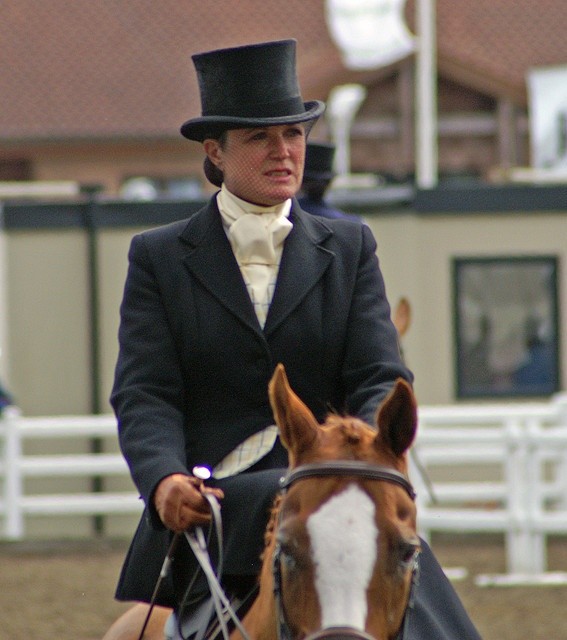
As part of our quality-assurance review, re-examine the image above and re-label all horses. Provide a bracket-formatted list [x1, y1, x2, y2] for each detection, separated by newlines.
[98, 361, 421, 640]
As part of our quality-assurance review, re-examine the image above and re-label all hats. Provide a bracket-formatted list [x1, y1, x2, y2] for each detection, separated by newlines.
[180, 38, 327, 143]
[303, 142, 338, 180]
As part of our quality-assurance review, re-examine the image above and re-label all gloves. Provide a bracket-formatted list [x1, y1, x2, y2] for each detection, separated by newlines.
[153, 473, 225, 532]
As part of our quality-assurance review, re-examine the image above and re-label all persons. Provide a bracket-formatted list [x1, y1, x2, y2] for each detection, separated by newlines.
[109, 37, 482, 639]
